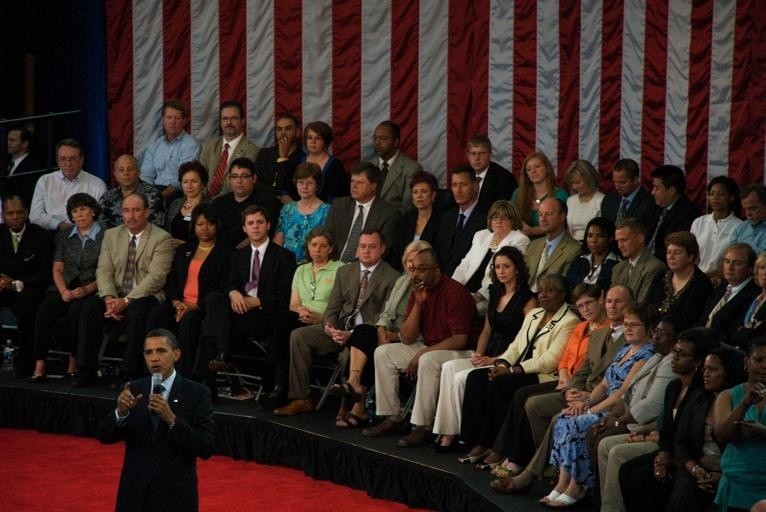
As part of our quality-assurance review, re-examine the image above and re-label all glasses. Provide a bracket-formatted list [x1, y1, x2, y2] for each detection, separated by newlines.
[409, 266, 435, 273]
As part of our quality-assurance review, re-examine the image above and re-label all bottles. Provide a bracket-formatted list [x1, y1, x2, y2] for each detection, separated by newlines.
[2, 340, 16, 373]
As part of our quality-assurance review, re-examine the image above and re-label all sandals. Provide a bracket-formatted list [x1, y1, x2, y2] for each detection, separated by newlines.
[328, 381, 589, 506]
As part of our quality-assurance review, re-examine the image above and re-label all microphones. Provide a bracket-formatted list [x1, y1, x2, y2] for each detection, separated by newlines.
[149, 372, 164, 412]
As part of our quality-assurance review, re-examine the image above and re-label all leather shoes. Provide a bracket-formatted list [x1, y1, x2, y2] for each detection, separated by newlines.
[65, 369, 81, 386]
[29, 374, 47, 383]
[208, 353, 236, 372]
[272, 399, 315, 416]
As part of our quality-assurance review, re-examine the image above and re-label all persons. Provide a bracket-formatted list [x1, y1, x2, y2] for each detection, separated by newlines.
[1, 101, 766, 512]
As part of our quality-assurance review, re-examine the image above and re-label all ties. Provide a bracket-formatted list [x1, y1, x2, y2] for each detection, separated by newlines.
[7, 161, 15, 176]
[12, 235, 19, 253]
[207, 143, 230, 199]
[245, 250, 261, 293]
[340, 205, 365, 262]
[151, 386, 165, 433]
[377, 161, 389, 197]
[123, 236, 137, 295]
[345, 271, 369, 329]
